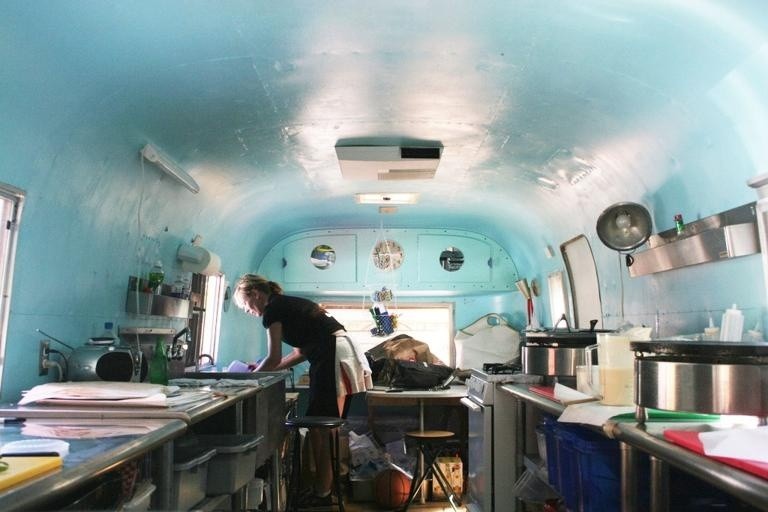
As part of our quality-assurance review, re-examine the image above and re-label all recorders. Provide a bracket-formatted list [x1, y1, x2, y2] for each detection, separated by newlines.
[67, 337, 149, 383]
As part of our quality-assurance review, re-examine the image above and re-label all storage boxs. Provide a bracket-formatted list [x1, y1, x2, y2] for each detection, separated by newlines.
[531, 413, 738, 511]
[432, 456, 464, 502]
[350, 480, 376, 503]
[53, 433, 264, 512]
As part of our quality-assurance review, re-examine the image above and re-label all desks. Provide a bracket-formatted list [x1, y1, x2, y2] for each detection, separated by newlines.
[366, 385, 469, 506]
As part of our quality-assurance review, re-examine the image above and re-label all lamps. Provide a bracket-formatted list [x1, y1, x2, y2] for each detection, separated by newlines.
[595, 201, 653, 252]
[138, 143, 200, 194]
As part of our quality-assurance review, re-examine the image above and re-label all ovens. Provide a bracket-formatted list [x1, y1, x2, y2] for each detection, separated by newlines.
[461, 396, 535, 512]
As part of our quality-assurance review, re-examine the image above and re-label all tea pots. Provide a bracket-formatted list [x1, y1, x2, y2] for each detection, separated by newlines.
[583, 328, 653, 407]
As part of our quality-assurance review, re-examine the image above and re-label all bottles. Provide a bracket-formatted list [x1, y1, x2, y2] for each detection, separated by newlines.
[102, 321, 120, 346]
[674, 213, 688, 240]
[146, 258, 167, 293]
[705, 301, 765, 344]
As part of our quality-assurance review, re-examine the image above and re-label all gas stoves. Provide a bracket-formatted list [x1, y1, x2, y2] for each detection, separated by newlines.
[465, 362, 536, 405]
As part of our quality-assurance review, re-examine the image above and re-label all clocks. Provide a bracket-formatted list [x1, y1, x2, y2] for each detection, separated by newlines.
[372, 239, 404, 271]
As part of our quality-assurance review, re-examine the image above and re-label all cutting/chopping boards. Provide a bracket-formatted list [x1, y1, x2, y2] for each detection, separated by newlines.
[0, 456, 63, 491]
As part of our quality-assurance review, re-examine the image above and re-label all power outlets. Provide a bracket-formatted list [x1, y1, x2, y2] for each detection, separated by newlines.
[38, 340, 50, 376]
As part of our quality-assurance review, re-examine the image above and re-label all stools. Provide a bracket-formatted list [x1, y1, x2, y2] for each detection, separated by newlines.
[284, 415, 346, 511]
[400, 430, 462, 512]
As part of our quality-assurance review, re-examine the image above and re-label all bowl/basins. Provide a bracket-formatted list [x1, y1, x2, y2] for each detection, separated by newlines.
[228, 360, 252, 373]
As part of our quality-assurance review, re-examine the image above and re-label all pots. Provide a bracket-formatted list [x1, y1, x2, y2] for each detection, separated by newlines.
[519, 327, 613, 378]
[627, 340, 768, 430]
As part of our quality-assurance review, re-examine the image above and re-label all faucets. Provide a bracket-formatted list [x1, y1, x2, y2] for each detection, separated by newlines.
[173, 327, 193, 360]
[196, 354, 214, 373]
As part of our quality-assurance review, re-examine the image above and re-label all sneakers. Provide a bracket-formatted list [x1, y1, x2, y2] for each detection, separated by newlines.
[298, 493, 332, 506]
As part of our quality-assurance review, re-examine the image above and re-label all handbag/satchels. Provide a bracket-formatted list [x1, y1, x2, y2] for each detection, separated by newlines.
[453, 314, 522, 371]
[387, 358, 457, 391]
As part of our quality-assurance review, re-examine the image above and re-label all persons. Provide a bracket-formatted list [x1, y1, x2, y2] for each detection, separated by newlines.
[233, 275, 374, 508]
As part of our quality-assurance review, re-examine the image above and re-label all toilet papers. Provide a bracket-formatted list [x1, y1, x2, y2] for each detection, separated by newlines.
[181, 245, 221, 275]
